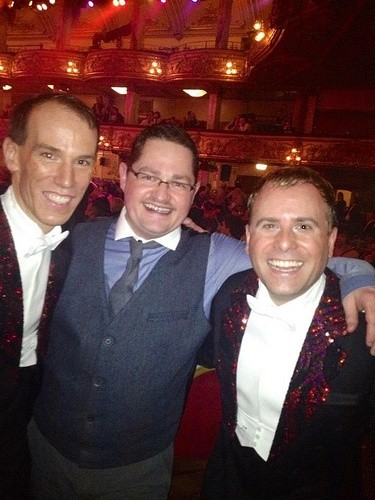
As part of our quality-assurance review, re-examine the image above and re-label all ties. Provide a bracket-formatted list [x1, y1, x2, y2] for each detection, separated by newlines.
[108, 240, 162, 316]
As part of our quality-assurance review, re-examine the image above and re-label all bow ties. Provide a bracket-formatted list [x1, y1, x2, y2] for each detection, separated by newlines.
[247, 294, 306, 331]
[23, 230, 70, 258]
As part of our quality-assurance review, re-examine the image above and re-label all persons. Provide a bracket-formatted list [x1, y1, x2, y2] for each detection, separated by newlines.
[0, 94, 101, 500]
[336, 191, 361, 234]
[194, 181, 247, 240]
[274, 113, 294, 135]
[27, 126, 375, 500]
[185, 110, 198, 126]
[89, 96, 124, 123]
[194, 167, 375, 500]
[138, 109, 162, 128]
[237, 114, 257, 133]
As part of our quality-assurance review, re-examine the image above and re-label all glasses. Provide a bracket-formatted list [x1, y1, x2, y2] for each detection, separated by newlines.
[128, 169, 196, 194]
